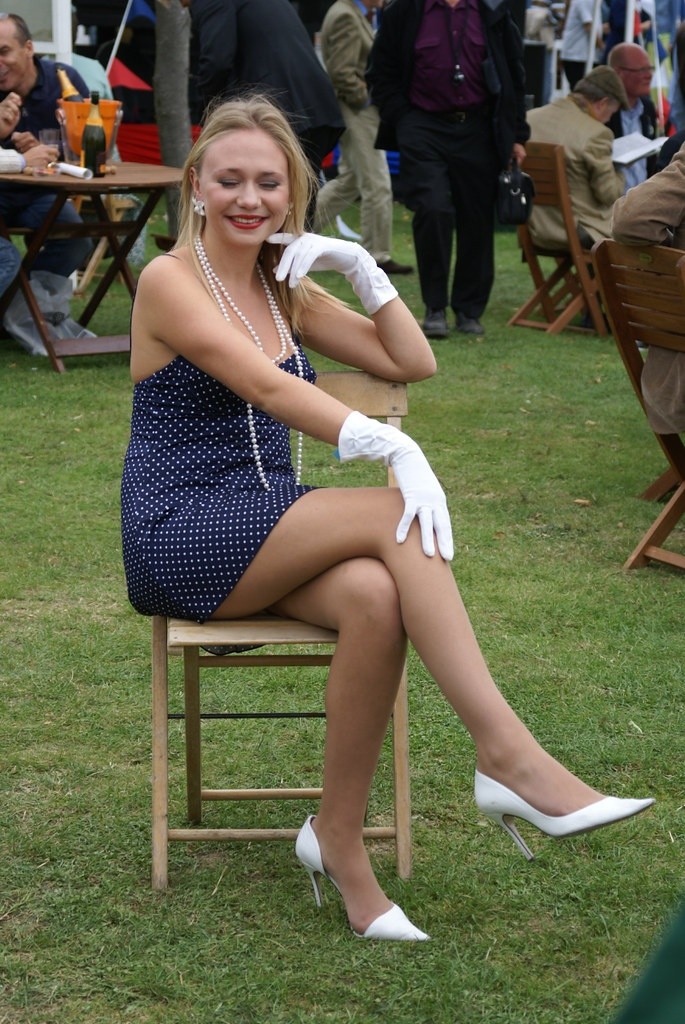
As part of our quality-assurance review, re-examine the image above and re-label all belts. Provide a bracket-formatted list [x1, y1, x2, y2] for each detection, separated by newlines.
[406, 102, 490, 125]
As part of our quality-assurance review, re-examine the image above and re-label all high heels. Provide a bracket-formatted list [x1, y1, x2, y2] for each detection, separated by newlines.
[295, 815, 431, 942]
[474, 769, 656, 863]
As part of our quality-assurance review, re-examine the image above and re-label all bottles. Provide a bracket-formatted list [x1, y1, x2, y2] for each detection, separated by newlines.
[80, 90, 106, 177]
[56, 66, 84, 104]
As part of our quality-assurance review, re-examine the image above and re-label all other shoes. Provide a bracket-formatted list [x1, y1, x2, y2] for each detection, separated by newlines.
[583, 310, 609, 329]
[454, 312, 485, 337]
[424, 308, 448, 337]
[377, 260, 414, 275]
[634, 340, 648, 348]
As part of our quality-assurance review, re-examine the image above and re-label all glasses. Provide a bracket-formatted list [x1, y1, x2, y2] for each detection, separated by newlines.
[615, 65, 654, 74]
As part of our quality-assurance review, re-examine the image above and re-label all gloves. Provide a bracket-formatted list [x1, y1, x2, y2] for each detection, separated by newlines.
[265, 230, 398, 315]
[337, 412, 455, 561]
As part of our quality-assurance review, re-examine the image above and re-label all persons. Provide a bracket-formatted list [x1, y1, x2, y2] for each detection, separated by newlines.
[525, 0, 651, 91]
[364, 0, 531, 337]
[318, 0, 412, 275]
[118, 96, 656, 941]
[176, 0, 348, 232]
[605, 44, 660, 196]
[516, 63, 628, 329]
[0, 10, 123, 358]
[610, 23, 685, 436]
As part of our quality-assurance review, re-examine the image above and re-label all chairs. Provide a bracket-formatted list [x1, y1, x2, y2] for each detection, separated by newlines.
[505, 142, 608, 335]
[149, 370, 412, 892]
[590, 238, 685, 580]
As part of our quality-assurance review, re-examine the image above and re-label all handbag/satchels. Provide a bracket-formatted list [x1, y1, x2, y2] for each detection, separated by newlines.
[497, 161, 535, 226]
[3, 270, 97, 356]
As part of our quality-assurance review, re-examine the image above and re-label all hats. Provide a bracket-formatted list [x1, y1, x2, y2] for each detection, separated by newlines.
[585, 65, 631, 113]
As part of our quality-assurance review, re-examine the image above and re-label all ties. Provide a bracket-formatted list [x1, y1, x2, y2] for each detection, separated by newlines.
[366, 11, 373, 26]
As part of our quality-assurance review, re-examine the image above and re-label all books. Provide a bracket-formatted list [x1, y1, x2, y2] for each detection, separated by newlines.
[608, 131, 669, 165]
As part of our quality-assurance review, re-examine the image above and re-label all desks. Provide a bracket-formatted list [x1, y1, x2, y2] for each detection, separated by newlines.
[1, 161, 185, 373]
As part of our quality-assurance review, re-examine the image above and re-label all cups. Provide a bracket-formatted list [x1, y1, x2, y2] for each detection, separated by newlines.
[39, 128, 60, 165]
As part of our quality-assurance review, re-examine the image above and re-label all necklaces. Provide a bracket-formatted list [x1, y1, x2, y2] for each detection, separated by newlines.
[194, 234, 308, 491]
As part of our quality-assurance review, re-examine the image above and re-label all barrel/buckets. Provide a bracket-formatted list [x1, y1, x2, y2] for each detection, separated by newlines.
[56, 96, 122, 167]
